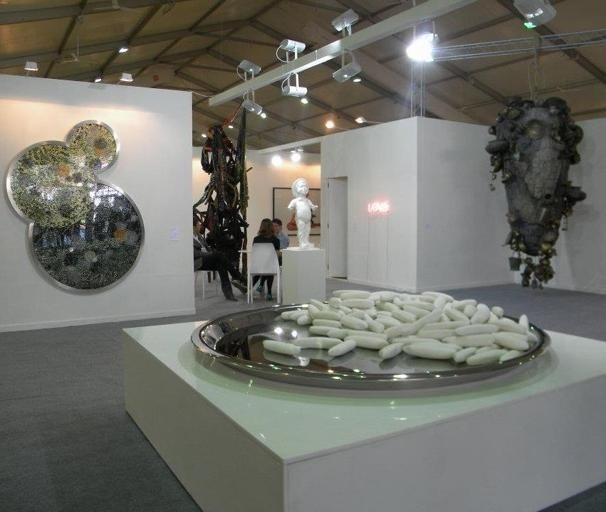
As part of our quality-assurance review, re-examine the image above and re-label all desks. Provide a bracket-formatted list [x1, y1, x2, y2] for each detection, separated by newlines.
[238, 249, 284, 299]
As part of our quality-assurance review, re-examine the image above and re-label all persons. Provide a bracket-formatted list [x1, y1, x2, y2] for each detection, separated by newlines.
[193, 216, 288, 301]
[287, 176, 318, 247]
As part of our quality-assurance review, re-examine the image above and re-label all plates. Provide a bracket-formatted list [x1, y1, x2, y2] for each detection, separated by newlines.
[191, 301, 551, 387]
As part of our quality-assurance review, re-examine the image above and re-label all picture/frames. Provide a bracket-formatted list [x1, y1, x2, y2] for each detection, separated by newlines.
[273, 187, 321, 236]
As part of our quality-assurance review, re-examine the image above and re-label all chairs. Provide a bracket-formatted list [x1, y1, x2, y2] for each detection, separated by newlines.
[247, 242, 281, 304]
[195, 270, 222, 301]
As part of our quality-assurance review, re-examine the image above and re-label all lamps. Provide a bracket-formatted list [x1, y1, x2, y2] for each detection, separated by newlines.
[208, 0, 489, 119]
[94, 70, 105, 83]
[118, 44, 130, 54]
[355, 116, 385, 124]
[513, 0, 557, 29]
[289, 145, 304, 163]
[24, 62, 39, 77]
[120, 73, 134, 83]
